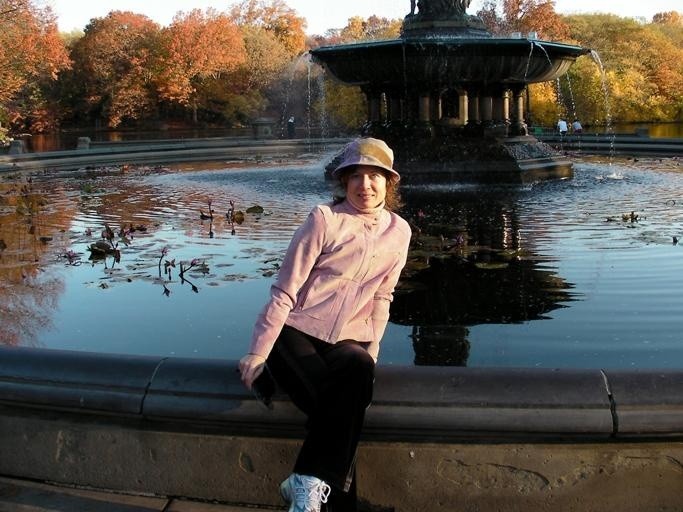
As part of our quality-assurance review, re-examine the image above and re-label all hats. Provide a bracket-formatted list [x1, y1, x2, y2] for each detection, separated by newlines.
[330, 136, 402, 186]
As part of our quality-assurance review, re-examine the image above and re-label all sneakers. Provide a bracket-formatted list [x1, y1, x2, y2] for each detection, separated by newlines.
[278, 470, 332, 512]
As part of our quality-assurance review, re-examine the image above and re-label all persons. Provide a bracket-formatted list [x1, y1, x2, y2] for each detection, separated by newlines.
[234, 134, 412, 512]
[286, 115, 296, 139]
[557, 118, 567, 142]
[571, 119, 584, 135]
